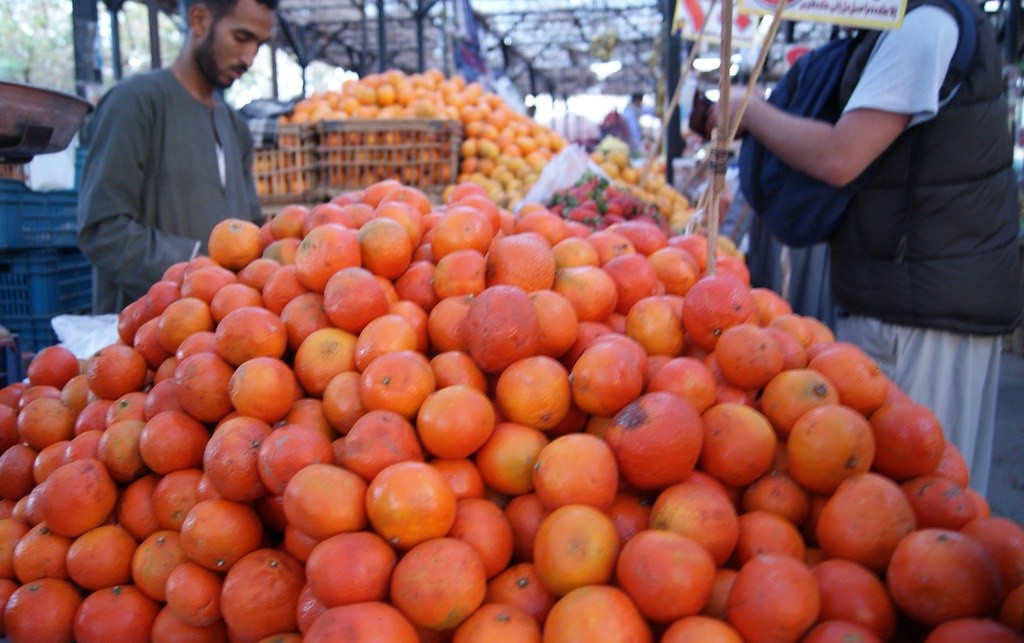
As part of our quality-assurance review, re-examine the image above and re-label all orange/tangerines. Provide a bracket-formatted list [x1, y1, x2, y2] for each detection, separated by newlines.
[0, 338, 1024, 643]
[554, 218, 833, 351]
[113, 178, 553, 352]
[589, 150, 742, 263]
[253, 67, 562, 205]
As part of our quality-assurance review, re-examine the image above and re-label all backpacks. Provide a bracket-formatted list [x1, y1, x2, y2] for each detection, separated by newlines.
[737, 1, 979, 250]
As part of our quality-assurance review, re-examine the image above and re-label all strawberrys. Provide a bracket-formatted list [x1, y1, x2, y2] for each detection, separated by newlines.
[547, 170, 666, 235]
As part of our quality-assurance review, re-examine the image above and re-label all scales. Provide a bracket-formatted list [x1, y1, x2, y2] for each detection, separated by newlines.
[0, 81, 95, 164]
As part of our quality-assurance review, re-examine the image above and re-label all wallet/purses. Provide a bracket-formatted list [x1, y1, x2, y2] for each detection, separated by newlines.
[690, 89, 746, 140]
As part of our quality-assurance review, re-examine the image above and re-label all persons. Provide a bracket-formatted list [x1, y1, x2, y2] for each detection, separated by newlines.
[710, 0, 1024, 501]
[621, 91, 655, 156]
[76, 0, 280, 316]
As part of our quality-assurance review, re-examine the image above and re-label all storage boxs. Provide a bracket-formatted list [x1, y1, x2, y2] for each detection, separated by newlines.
[0, 178, 79, 250]
[0, 318, 63, 384]
[0, 248, 92, 320]
[248, 123, 319, 214]
[316, 118, 463, 198]
